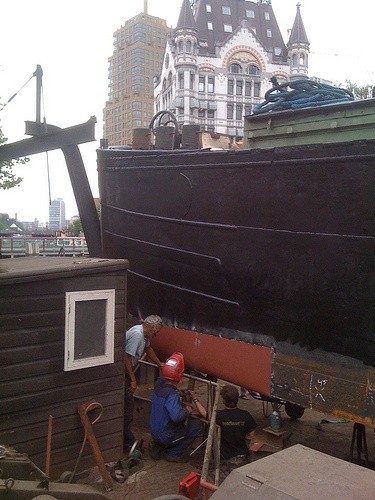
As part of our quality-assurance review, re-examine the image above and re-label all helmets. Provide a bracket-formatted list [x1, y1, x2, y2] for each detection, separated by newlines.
[162, 352, 184, 382]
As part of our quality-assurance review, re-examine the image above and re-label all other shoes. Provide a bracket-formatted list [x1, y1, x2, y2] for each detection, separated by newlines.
[150, 447, 161, 460]
[166, 455, 188, 463]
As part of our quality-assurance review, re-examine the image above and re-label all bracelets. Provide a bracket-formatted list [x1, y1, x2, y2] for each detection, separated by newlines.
[193, 398, 199, 404]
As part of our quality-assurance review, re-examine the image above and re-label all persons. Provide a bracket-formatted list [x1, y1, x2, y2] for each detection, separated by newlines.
[150, 351, 204, 461]
[111, 315, 162, 453]
[188, 385, 257, 461]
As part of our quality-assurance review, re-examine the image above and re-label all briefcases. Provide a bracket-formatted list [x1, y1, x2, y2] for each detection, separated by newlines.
[178, 471, 201, 498]
